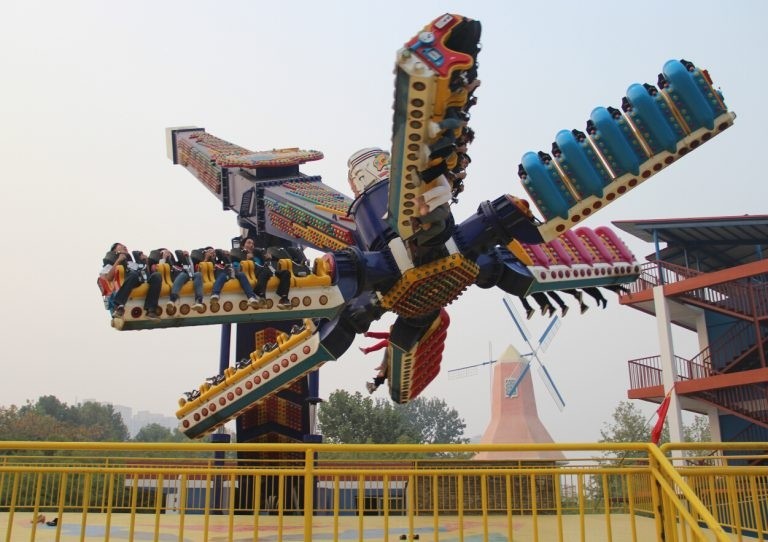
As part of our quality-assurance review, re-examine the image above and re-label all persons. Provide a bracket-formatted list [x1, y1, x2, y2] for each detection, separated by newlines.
[521, 284, 632, 320]
[100, 237, 292, 321]
[359, 324, 395, 395]
[412, 100, 473, 251]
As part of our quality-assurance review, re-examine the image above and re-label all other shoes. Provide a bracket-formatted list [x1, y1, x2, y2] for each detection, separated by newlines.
[526, 309, 535, 320]
[602, 299, 607, 309]
[167, 301, 174, 312]
[626, 290, 633, 302]
[597, 297, 601, 306]
[364, 332, 368, 337]
[540, 304, 548, 316]
[146, 311, 160, 322]
[112, 305, 125, 317]
[358, 347, 368, 355]
[259, 298, 267, 305]
[421, 145, 431, 164]
[428, 121, 441, 138]
[367, 383, 376, 394]
[416, 218, 424, 227]
[283, 296, 291, 306]
[411, 236, 420, 248]
[373, 378, 384, 385]
[411, 169, 422, 186]
[549, 308, 556, 317]
[561, 306, 569, 317]
[211, 296, 219, 303]
[248, 298, 259, 306]
[580, 304, 587, 313]
[191, 300, 204, 310]
